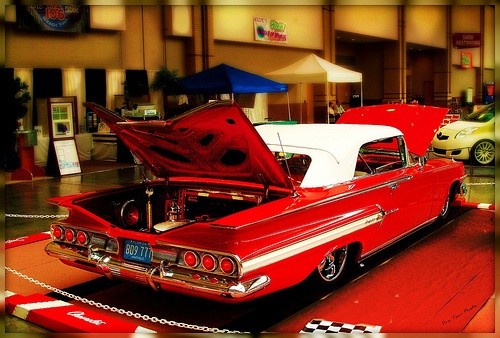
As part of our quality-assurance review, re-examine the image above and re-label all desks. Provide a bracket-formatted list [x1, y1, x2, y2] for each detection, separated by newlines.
[33, 133, 93, 167]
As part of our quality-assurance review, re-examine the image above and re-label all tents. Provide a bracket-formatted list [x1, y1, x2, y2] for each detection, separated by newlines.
[264, 54, 362, 124]
[163, 63, 291, 121]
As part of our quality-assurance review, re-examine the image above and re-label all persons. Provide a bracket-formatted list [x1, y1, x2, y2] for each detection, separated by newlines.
[329, 99, 345, 123]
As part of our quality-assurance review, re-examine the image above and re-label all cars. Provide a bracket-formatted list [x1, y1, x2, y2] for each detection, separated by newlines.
[431, 103, 496, 165]
[45, 100, 470, 310]
